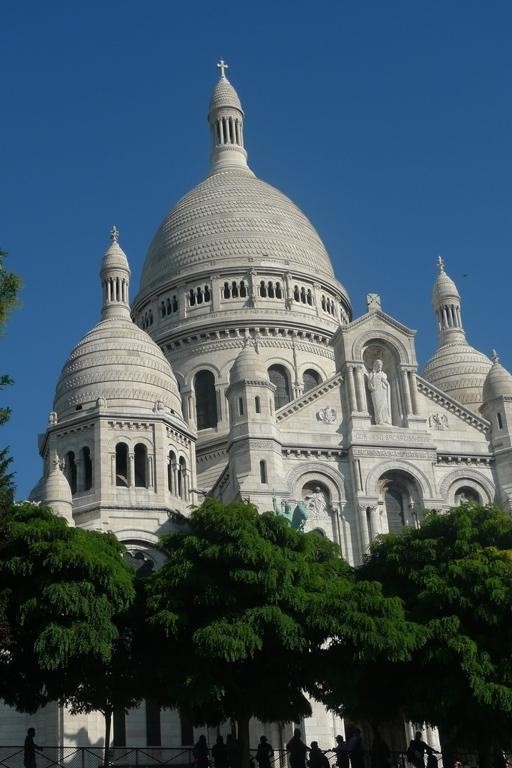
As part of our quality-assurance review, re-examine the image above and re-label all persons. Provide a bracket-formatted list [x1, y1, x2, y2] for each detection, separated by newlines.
[367, 359, 393, 425]
[193, 729, 461, 768]
[24, 728, 42, 768]
[306, 487, 326, 514]
